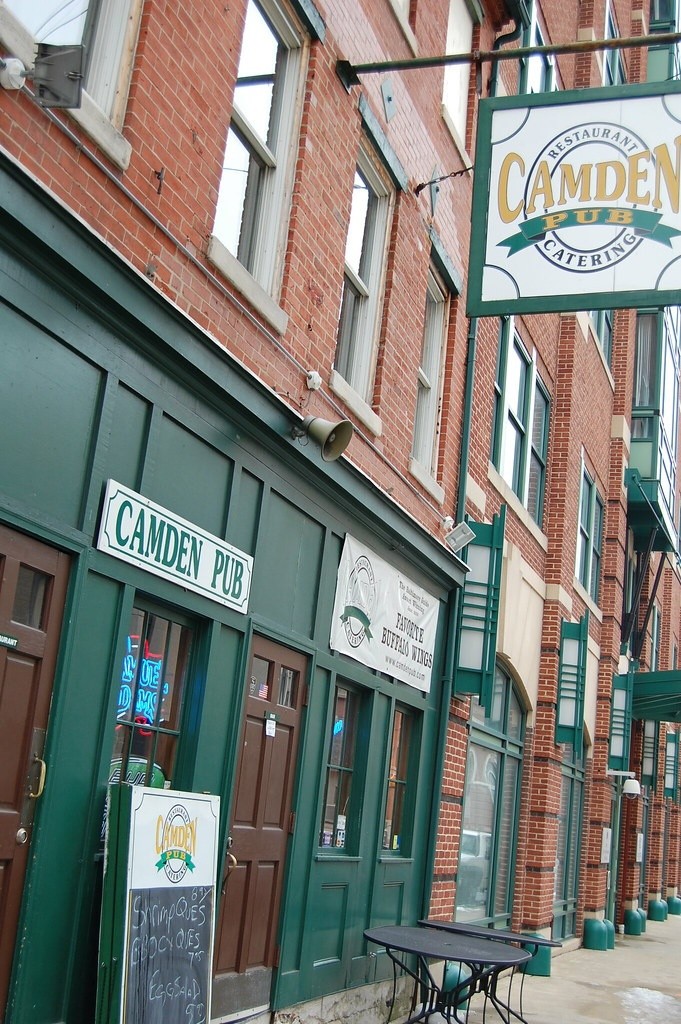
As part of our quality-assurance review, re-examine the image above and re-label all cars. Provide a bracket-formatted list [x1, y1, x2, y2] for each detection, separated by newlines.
[456, 829, 494, 907]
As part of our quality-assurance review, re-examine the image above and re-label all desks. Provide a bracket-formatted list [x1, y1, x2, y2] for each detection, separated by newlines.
[418, 918, 562, 1024]
[363, 924, 532, 1024]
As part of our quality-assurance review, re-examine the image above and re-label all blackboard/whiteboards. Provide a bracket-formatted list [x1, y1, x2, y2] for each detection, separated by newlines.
[123, 885, 215, 1024]
